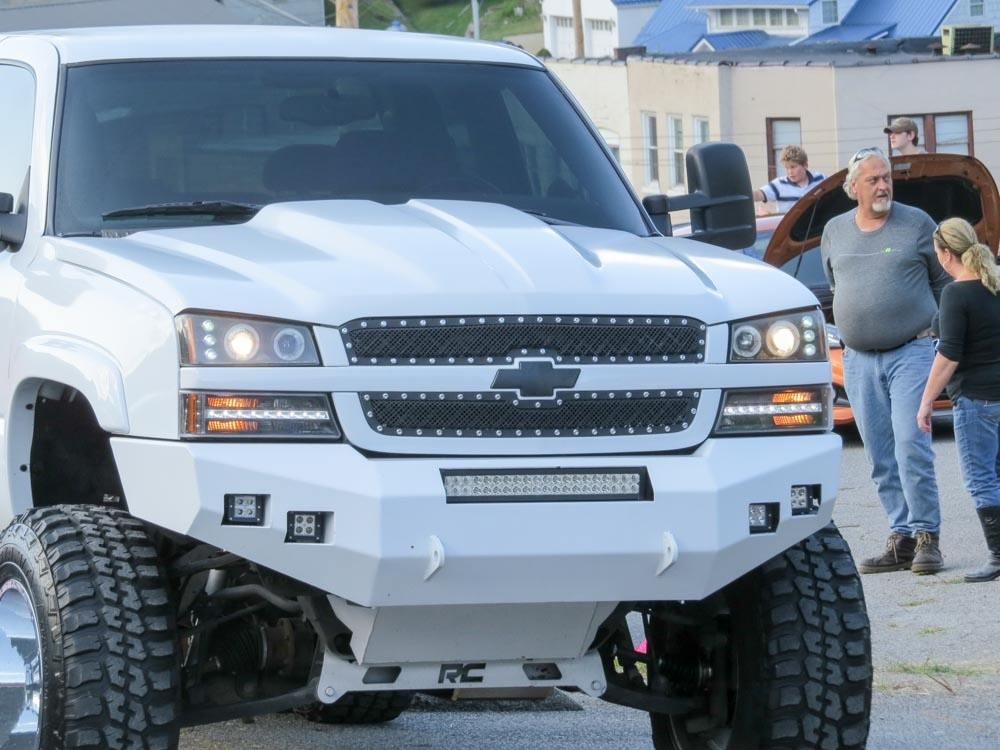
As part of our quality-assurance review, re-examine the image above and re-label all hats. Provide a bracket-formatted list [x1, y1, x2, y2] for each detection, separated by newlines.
[884, 117, 918, 134]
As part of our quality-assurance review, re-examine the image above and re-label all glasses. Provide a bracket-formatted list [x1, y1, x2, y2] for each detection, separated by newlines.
[854, 147, 884, 162]
[936, 219, 951, 248]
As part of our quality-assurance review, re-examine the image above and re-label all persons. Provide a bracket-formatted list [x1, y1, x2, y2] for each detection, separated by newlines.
[883, 117, 930, 154]
[750, 145, 826, 215]
[916, 217, 1000, 582]
[820, 145, 955, 574]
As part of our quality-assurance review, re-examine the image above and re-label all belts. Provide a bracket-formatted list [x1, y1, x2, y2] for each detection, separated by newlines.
[867, 329, 928, 352]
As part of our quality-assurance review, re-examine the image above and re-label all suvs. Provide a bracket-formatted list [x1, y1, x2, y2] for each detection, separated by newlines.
[0, 25, 872, 750]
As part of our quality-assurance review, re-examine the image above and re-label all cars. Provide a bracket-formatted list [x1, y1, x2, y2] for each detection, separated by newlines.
[672, 153, 1000, 427]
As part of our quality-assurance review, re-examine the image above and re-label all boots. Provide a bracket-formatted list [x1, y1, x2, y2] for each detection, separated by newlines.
[860, 533, 917, 573]
[911, 530, 944, 574]
[964, 505, 1000, 581]
